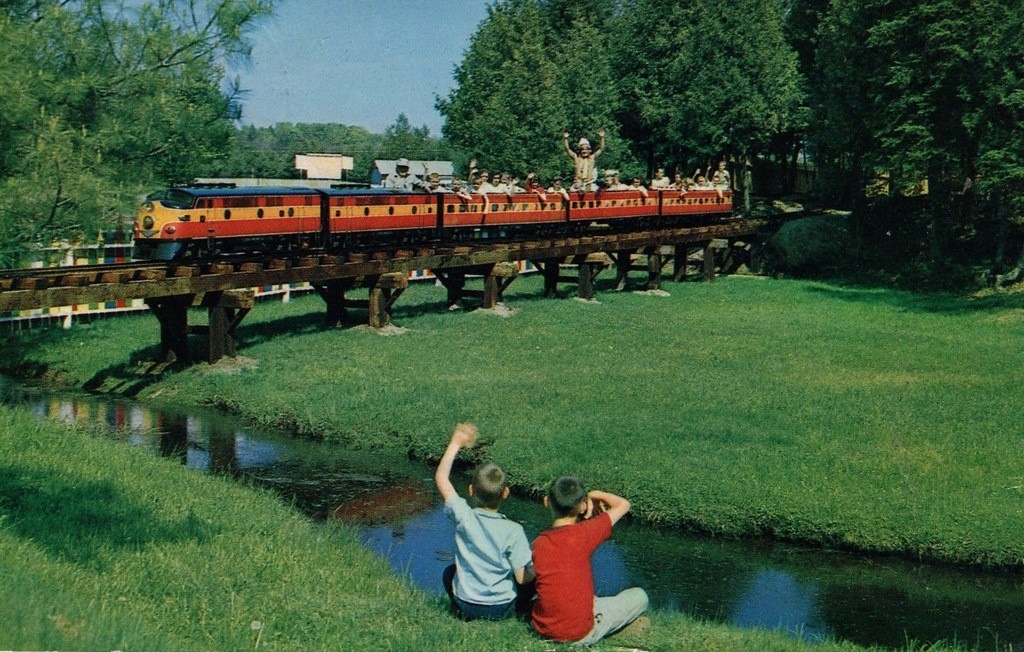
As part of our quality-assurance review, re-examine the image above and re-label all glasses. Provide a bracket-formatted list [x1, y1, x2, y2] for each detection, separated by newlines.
[493, 177, 500, 179]
[432, 181, 439, 184]
[481, 176, 489, 178]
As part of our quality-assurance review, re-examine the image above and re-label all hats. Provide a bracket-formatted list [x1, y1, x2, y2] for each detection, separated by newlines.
[578, 137, 590, 148]
[395, 157, 409, 166]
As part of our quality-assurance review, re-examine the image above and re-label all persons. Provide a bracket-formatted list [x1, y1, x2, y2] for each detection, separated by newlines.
[530, 475, 649, 647]
[564, 127, 605, 192]
[435, 420, 534, 621]
[385, 158, 730, 214]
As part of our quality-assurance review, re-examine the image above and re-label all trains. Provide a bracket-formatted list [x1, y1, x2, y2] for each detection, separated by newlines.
[129, 177, 735, 266]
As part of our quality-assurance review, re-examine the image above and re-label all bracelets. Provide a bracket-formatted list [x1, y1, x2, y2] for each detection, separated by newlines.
[600, 136, 604, 138]
[564, 138, 568, 139]
